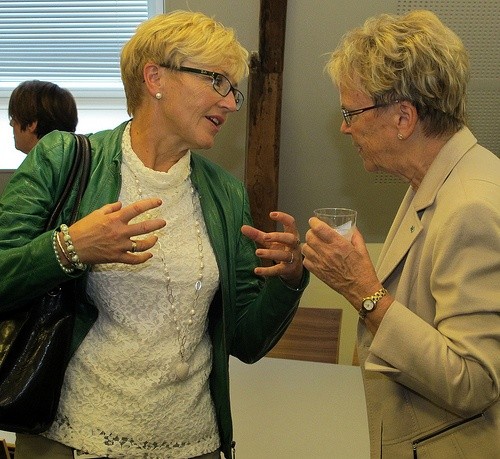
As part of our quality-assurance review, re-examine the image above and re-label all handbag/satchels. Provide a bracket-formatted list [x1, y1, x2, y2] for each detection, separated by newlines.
[0, 133, 99, 435]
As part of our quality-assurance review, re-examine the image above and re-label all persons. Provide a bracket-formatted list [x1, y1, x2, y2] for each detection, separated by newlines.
[0, 11, 309, 458]
[7, 80, 78, 155]
[301, 10, 500, 458]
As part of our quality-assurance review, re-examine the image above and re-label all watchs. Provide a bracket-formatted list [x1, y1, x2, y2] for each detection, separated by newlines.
[358, 289, 388, 320]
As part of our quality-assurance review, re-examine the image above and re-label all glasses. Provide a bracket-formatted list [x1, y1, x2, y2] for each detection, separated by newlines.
[341, 99, 423, 126]
[142, 65, 244, 110]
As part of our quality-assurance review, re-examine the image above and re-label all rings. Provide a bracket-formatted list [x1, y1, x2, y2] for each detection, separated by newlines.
[287, 253, 294, 264]
[295, 239, 301, 246]
[130, 240, 137, 253]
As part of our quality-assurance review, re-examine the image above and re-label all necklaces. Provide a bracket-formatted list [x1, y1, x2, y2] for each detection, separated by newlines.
[122, 129, 205, 381]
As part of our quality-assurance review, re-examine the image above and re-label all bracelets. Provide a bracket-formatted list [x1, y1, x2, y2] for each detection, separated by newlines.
[53, 224, 86, 273]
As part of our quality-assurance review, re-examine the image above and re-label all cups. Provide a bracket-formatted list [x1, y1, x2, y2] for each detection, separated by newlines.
[313, 208, 358, 244]
